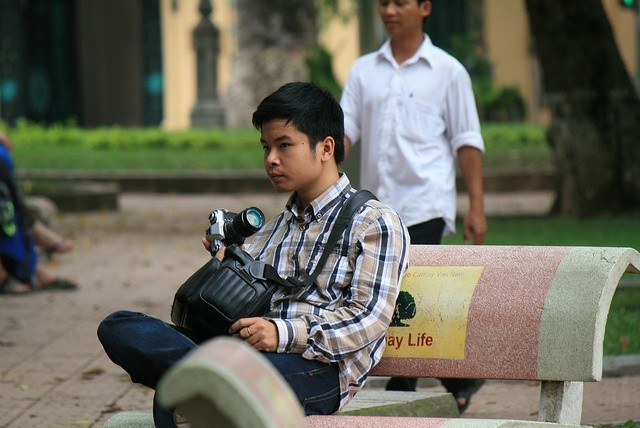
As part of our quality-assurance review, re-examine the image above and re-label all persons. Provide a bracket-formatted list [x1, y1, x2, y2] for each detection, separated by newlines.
[339, 0, 486, 415]
[1, 130, 80, 296]
[98, 81, 411, 416]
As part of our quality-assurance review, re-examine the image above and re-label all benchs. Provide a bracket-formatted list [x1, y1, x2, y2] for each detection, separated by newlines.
[102, 245, 639, 428]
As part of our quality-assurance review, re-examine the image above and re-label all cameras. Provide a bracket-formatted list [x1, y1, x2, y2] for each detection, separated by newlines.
[205, 206, 265, 257]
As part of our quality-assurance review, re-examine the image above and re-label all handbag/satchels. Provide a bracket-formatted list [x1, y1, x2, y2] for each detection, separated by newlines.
[171, 243, 280, 335]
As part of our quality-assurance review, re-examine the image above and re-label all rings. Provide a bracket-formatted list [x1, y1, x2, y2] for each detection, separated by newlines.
[246, 326, 253, 336]
